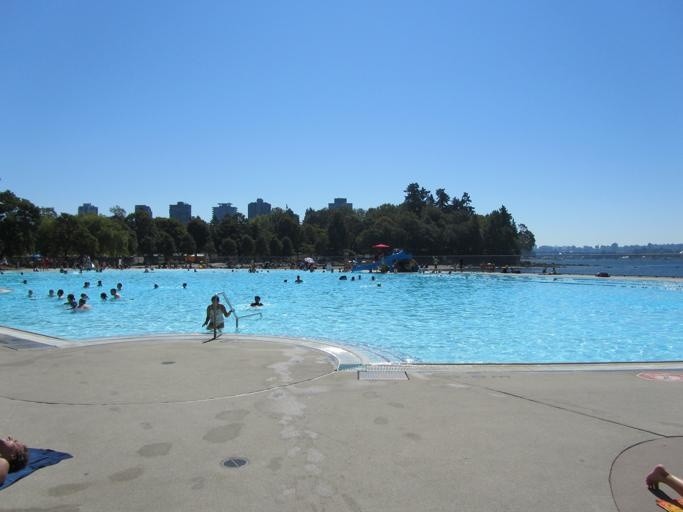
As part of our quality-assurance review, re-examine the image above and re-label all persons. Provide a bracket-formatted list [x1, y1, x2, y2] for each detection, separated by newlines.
[250, 295, 262, 306]
[27, 289, 32, 298]
[294, 275, 302, 284]
[200, 294, 235, 329]
[0, 434, 28, 489]
[644, 463, 682, 497]
[47, 280, 122, 313]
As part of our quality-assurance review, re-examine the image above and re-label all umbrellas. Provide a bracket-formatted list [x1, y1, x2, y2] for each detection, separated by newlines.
[304, 256, 315, 264]
[370, 243, 390, 249]
